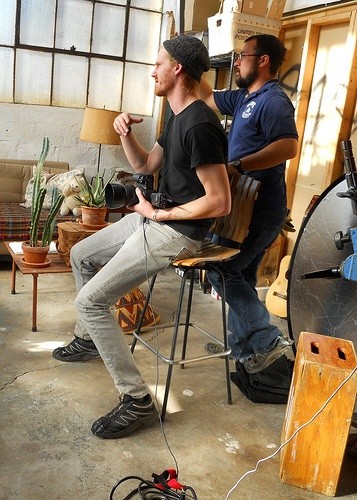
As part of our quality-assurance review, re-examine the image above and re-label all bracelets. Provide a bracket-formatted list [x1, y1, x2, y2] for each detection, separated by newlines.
[152, 209, 158, 221]
[117, 126, 132, 134]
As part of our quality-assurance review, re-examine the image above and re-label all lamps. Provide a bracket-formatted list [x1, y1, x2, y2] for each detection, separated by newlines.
[80, 105, 122, 189]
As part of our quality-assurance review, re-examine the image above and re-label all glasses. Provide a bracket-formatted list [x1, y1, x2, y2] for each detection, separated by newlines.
[239, 52, 264, 60]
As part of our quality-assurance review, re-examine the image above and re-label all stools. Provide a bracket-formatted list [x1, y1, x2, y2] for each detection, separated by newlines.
[131, 172, 261, 422]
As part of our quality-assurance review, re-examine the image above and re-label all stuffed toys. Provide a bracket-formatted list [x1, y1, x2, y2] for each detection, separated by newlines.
[60, 178, 84, 216]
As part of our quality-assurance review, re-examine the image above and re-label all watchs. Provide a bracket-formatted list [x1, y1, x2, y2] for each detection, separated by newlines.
[234, 159, 244, 173]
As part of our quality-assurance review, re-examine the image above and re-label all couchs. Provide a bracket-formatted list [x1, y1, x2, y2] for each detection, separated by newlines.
[0, 159, 82, 263]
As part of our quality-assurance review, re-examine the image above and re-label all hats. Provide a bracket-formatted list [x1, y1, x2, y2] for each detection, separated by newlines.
[163, 35, 210, 84]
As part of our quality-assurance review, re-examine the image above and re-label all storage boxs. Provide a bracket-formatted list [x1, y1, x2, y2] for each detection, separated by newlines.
[208, 11, 283, 57]
[281, 333, 357, 497]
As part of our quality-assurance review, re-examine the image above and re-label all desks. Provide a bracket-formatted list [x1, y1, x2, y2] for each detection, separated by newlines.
[4, 241, 74, 331]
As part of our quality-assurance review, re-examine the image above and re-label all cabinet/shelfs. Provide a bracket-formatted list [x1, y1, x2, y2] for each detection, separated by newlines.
[210, 51, 283, 136]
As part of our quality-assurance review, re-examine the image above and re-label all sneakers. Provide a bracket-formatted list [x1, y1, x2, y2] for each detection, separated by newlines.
[52, 333, 101, 361]
[245, 333, 293, 373]
[91, 394, 159, 439]
[205, 342, 235, 360]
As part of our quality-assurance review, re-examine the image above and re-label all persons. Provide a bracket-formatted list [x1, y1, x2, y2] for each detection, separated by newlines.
[195, 35, 300, 374]
[53, 35, 232, 440]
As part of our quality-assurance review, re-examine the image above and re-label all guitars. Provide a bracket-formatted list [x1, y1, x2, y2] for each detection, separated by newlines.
[264, 194, 325, 317]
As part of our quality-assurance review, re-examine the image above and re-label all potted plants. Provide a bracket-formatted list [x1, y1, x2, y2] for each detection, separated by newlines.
[74, 168, 117, 224]
[22, 136, 65, 263]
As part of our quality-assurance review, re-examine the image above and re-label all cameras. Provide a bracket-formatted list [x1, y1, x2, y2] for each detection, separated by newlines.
[105, 172, 173, 209]
